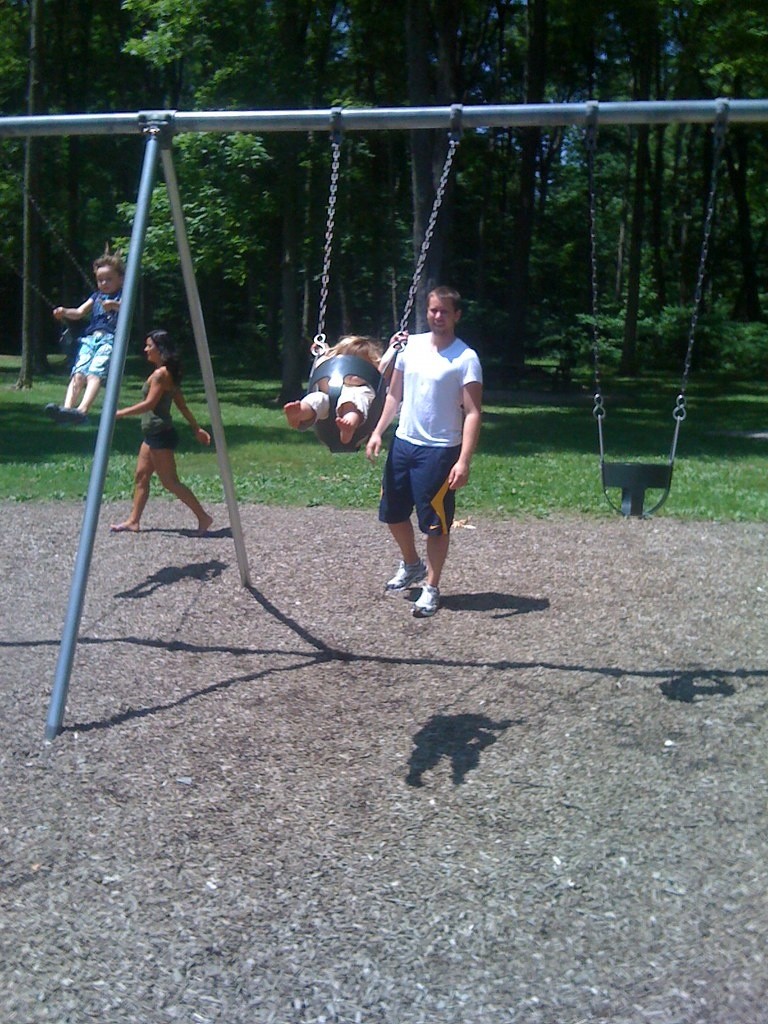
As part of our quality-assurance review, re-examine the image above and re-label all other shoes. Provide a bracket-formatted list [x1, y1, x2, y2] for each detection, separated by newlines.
[61, 406, 89, 424]
[44, 402, 65, 425]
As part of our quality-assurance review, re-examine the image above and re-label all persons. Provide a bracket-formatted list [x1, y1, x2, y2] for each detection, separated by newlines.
[284, 331, 409, 444]
[110, 332, 213, 536]
[46, 243, 123, 423]
[365, 286, 482, 617]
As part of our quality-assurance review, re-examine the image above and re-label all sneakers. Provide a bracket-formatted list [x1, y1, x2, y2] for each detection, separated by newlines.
[386, 558, 428, 591]
[411, 587, 440, 617]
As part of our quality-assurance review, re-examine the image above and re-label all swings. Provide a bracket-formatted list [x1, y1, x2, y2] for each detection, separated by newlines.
[306, 137, 461, 455]
[0, 152, 123, 388]
[586, 126, 727, 518]
